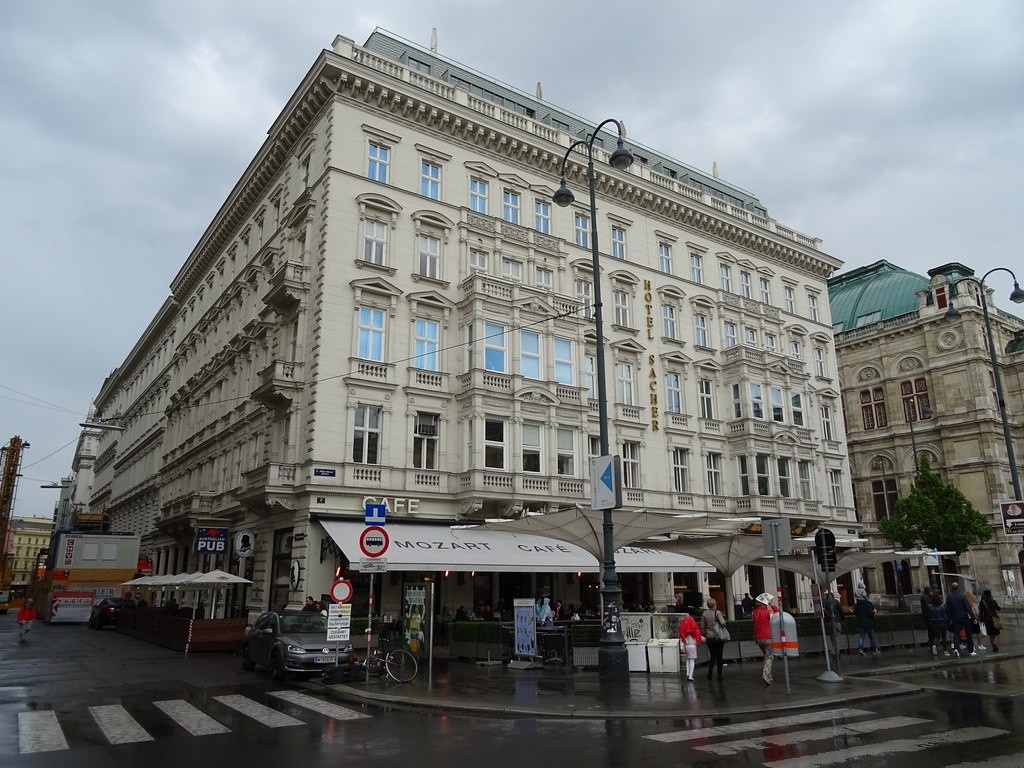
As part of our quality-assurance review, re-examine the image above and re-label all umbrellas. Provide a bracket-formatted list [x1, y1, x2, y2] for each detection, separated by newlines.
[119, 568, 254, 620]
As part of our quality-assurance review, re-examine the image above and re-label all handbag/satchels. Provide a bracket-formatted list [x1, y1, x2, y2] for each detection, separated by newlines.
[948, 621, 955, 633]
[713, 621, 731, 644]
[979, 622, 987, 636]
[992, 616, 1003, 630]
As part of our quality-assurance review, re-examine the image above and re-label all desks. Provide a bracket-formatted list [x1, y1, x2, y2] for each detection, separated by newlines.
[379, 622, 397, 644]
[536, 626, 572, 660]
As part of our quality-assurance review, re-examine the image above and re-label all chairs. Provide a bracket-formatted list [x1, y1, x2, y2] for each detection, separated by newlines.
[310, 621, 325, 632]
[378, 622, 394, 650]
[270, 618, 286, 633]
[502, 625, 566, 664]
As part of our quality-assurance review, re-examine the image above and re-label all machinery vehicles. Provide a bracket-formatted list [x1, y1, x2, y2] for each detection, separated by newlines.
[0, 436, 30, 614]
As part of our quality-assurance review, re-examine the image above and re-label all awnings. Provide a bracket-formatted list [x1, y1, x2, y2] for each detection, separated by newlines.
[307, 512, 718, 572]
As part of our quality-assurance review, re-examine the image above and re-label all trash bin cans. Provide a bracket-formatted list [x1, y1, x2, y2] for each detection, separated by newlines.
[769, 613, 799, 656]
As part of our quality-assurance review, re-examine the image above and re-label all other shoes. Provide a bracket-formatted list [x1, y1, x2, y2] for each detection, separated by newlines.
[21, 639, 26, 642]
[951, 643, 954, 649]
[992, 643, 999, 653]
[944, 651, 950, 656]
[859, 650, 865, 655]
[970, 652, 977, 655]
[959, 644, 966, 649]
[932, 645, 937, 655]
[717, 678, 723, 681]
[688, 676, 694, 681]
[954, 649, 959, 657]
[20, 633, 22, 639]
[873, 648, 881, 654]
[763, 678, 770, 686]
[978, 644, 986, 650]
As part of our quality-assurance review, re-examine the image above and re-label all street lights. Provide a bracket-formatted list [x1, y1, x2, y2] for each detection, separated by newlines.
[552, 119, 634, 678]
[944, 268, 1024, 586]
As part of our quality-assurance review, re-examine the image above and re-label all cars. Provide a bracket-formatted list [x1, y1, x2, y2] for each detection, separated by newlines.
[88, 598, 136, 629]
[245, 609, 353, 678]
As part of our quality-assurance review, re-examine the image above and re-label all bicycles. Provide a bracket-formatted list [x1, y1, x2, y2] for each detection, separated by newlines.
[354, 646, 418, 683]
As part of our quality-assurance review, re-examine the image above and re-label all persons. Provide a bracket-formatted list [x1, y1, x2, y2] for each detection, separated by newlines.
[17, 598, 36, 643]
[456, 596, 660, 625]
[678, 581, 1002, 685]
[306, 596, 318, 611]
